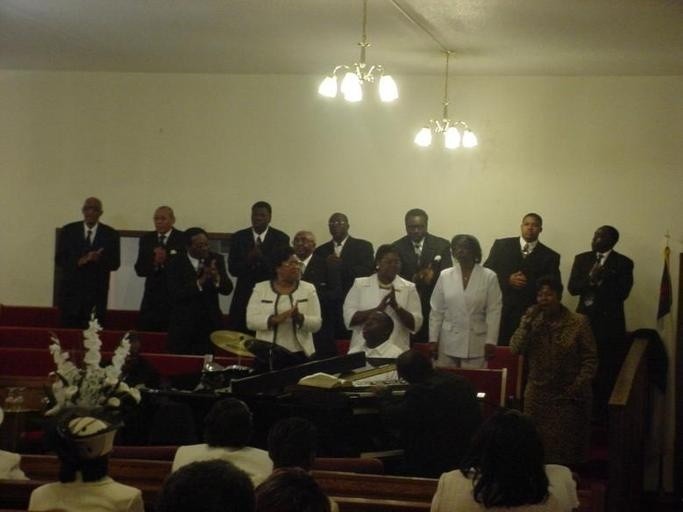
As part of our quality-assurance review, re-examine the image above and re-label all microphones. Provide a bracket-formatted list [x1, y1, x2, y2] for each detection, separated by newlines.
[525, 303, 542, 325]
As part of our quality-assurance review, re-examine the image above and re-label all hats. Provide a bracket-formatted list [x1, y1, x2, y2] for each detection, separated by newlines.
[56, 409, 119, 461]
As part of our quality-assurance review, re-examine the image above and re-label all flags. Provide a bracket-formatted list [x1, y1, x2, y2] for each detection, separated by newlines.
[657, 248, 673, 321]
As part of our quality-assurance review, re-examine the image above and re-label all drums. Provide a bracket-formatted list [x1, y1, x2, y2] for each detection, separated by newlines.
[201, 365, 253, 388]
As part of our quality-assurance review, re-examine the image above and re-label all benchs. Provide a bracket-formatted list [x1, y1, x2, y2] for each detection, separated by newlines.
[0, 298, 525, 511]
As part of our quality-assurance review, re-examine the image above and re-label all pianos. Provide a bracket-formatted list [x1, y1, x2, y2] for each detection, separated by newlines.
[297, 372, 411, 458]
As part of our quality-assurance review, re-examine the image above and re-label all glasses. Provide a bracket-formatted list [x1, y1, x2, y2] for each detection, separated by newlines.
[329, 220, 348, 226]
[279, 260, 304, 267]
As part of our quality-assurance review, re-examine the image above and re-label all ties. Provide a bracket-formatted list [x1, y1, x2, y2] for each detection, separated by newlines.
[159, 235, 166, 245]
[85, 230, 92, 247]
[256, 236, 262, 249]
[596, 254, 605, 265]
[414, 243, 423, 255]
[523, 244, 530, 254]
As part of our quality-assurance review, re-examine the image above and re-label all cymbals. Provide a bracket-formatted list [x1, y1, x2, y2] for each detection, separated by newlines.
[244, 339, 295, 366]
[210, 330, 257, 357]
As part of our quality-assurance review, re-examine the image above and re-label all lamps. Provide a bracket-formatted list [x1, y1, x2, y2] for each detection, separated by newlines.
[413, 51, 478, 150]
[315, 0, 399, 109]
